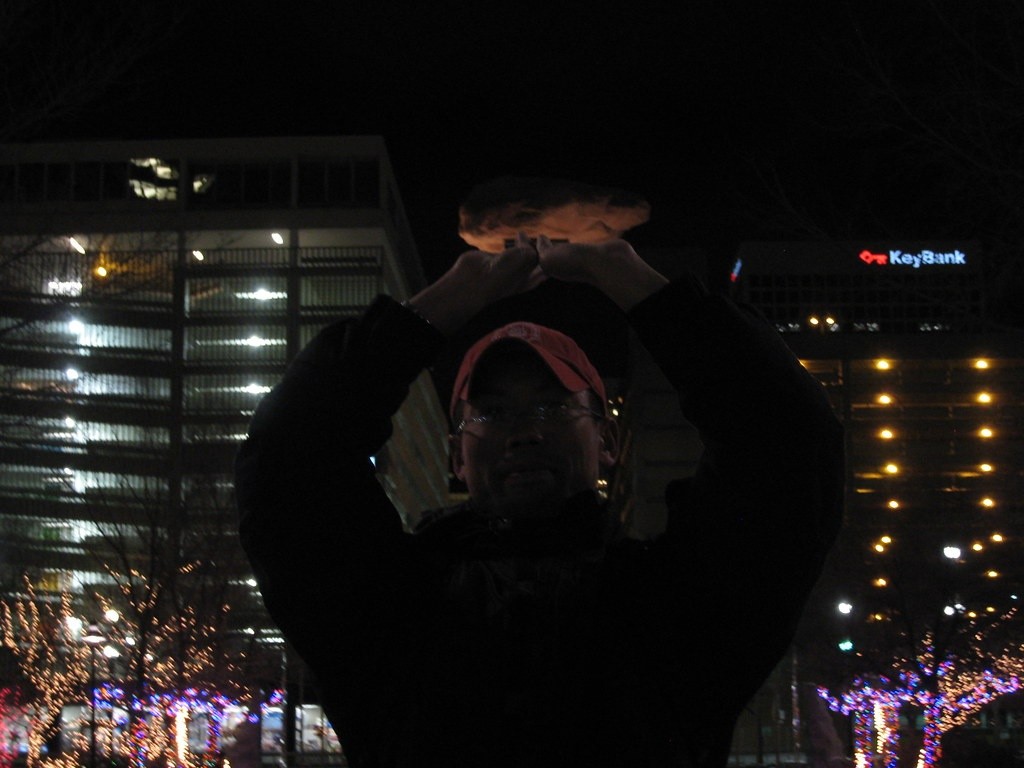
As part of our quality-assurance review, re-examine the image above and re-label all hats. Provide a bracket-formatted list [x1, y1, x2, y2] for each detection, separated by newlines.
[451, 322, 608, 411]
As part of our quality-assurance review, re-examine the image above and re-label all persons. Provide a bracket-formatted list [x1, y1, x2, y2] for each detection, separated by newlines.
[234, 231, 844, 768]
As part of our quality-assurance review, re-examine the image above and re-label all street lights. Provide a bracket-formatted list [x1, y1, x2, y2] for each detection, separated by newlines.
[126, 143, 211, 592]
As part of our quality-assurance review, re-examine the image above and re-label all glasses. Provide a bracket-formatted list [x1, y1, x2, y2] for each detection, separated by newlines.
[451, 397, 605, 439]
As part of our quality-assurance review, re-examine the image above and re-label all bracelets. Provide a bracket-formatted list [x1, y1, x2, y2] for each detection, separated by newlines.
[401, 299, 433, 326]
